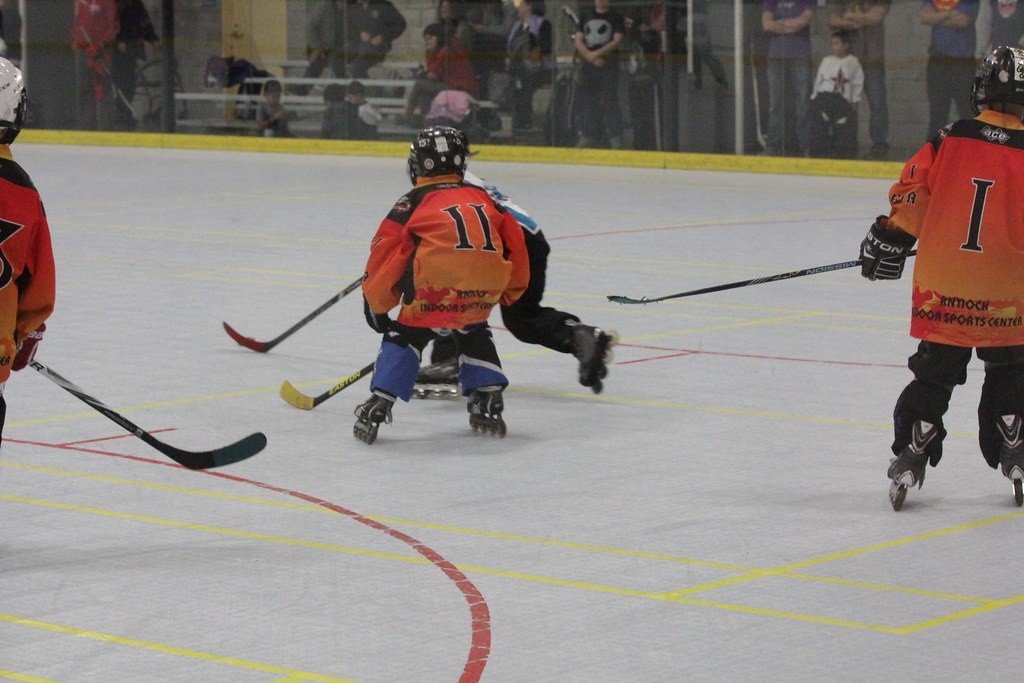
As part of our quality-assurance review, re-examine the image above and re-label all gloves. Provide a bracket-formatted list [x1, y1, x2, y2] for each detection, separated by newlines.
[363, 295, 393, 333]
[12, 323, 50, 372]
[83, 41, 109, 59]
[859, 215, 917, 281]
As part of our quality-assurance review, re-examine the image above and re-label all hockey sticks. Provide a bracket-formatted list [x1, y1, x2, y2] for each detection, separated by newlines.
[25, 357, 270, 471]
[221, 276, 363, 354]
[604, 246, 918, 306]
[79, 26, 142, 120]
[278, 361, 376, 412]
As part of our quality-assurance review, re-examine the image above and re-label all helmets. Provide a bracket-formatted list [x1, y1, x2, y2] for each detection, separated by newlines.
[0, 57, 27, 144]
[969, 45, 1024, 116]
[408, 124, 468, 186]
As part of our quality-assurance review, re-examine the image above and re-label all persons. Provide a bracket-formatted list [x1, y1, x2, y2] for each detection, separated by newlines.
[0, 57, 56, 445]
[412, 171, 606, 393]
[69, 0, 1024, 167]
[352, 126, 507, 445]
[859, 46, 1024, 488]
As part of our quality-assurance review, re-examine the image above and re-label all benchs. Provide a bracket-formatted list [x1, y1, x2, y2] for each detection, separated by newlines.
[173, 59, 523, 138]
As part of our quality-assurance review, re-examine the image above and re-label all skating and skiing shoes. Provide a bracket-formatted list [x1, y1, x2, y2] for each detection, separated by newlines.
[996, 415, 1024, 506]
[564, 319, 617, 395]
[887, 420, 939, 511]
[467, 385, 512, 438]
[353, 390, 397, 444]
[416, 358, 460, 398]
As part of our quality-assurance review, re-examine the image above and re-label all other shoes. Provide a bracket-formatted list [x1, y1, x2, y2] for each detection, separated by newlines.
[73, 91, 137, 131]
[758, 146, 809, 157]
[870, 142, 888, 150]
[388, 112, 427, 127]
[575, 137, 624, 149]
